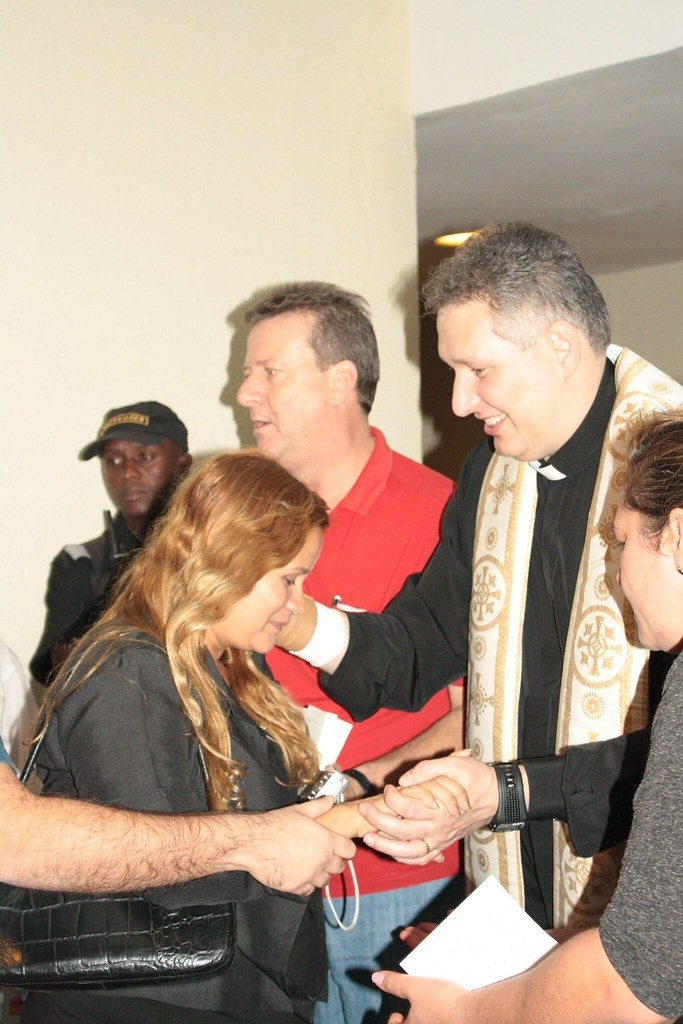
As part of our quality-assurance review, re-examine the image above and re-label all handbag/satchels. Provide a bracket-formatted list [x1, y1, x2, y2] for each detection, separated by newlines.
[0, 638, 236, 992]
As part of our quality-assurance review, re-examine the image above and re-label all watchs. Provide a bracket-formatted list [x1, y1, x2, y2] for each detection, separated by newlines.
[493, 763, 528, 833]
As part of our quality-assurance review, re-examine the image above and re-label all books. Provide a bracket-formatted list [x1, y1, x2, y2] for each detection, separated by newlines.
[396, 874, 560, 991]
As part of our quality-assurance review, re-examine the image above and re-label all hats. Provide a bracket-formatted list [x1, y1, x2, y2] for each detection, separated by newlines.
[81, 402, 187, 460]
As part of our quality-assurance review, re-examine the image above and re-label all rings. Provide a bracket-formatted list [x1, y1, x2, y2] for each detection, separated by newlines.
[421, 839, 430, 853]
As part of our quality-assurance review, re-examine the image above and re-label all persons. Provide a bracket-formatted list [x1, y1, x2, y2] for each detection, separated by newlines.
[372, 413, 683, 1024]
[27, 402, 195, 690]
[0, 450, 467, 1024]
[237, 277, 465, 1024]
[0, 762, 353, 897]
[274, 219, 683, 932]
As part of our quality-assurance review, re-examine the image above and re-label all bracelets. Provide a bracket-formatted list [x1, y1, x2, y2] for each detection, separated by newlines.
[345, 769, 375, 794]
